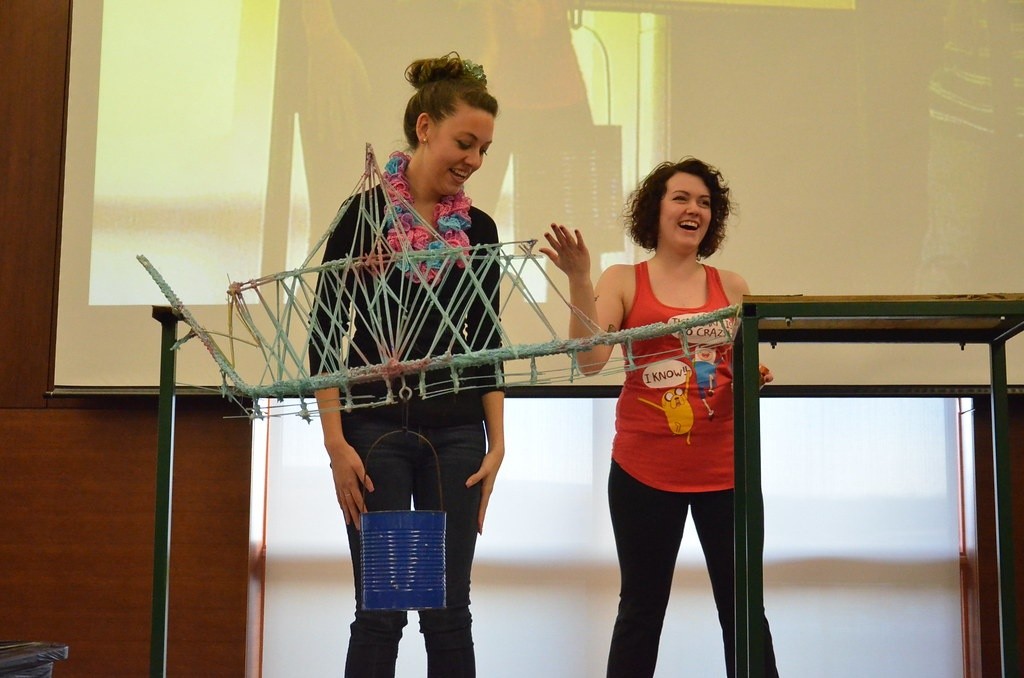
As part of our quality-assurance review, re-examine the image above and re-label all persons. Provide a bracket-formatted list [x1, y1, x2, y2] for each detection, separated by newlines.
[307, 51, 506, 678]
[538, 157, 780, 678]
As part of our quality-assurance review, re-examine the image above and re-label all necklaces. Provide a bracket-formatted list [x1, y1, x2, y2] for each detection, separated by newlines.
[380, 151, 472, 289]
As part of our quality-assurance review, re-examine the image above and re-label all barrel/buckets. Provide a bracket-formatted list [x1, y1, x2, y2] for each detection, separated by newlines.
[360, 430, 450, 612]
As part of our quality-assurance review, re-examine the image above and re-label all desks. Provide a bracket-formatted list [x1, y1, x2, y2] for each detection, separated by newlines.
[732, 293, 1024, 678]
